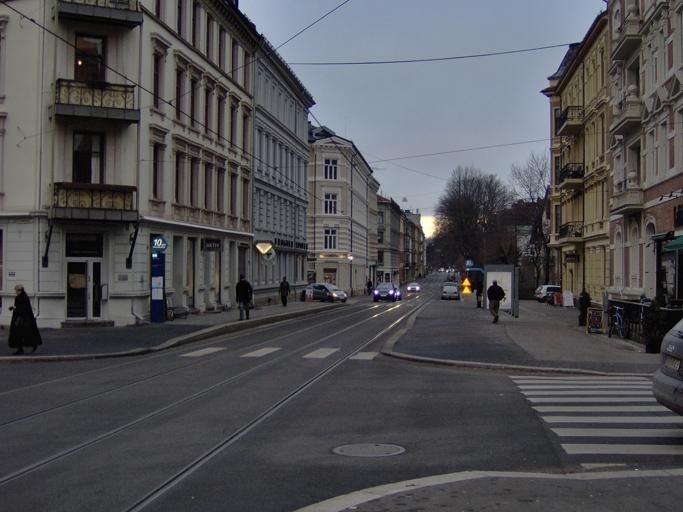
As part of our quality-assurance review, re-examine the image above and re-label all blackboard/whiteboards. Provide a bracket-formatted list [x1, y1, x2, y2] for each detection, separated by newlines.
[587, 308, 605, 330]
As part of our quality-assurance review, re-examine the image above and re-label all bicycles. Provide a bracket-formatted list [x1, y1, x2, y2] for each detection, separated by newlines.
[607, 304, 630, 339]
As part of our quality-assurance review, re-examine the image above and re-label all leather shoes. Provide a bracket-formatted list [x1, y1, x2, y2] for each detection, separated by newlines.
[493, 315, 499, 324]
[12, 347, 38, 356]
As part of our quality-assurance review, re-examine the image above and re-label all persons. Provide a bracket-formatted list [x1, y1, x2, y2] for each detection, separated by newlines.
[236, 273, 252, 321]
[6, 284, 44, 355]
[366, 278, 372, 296]
[279, 276, 290, 307]
[487, 280, 505, 322]
[476, 277, 484, 308]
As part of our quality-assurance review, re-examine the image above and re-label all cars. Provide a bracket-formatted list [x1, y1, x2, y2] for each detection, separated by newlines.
[407, 283, 421, 292]
[534, 285, 561, 303]
[373, 281, 402, 302]
[652, 318, 683, 418]
[440, 282, 460, 300]
[305, 283, 349, 303]
